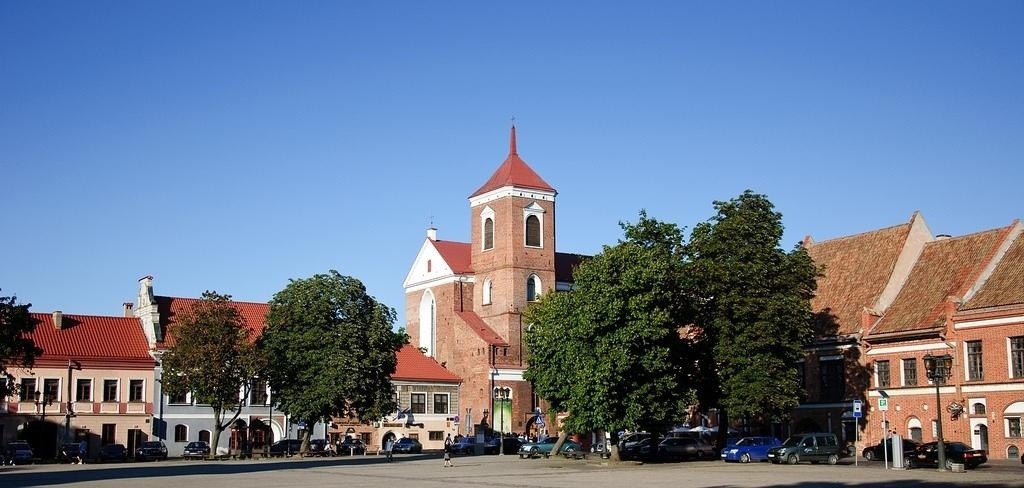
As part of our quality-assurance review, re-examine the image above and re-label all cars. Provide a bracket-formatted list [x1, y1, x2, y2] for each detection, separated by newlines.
[495, 438, 527, 454]
[590, 441, 604, 453]
[453, 437, 498, 455]
[517, 437, 581, 458]
[393, 438, 422, 454]
[59, 443, 80, 463]
[338, 439, 367, 455]
[100, 444, 128, 462]
[309, 440, 324, 450]
[863, 437, 920, 461]
[904, 441, 987, 469]
[618, 431, 717, 460]
[184, 441, 210, 460]
[138, 440, 168, 461]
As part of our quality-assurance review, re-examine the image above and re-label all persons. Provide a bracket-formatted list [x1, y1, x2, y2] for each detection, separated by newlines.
[503, 430, 549, 443]
[246, 432, 254, 458]
[62, 436, 88, 464]
[385, 439, 392, 461]
[401, 433, 405, 438]
[444, 445, 453, 467]
[325, 435, 333, 456]
[888, 430, 896, 439]
[444, 433, 452, 445]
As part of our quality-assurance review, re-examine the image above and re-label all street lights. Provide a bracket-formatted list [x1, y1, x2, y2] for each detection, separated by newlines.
[34, 390, 48, 463]
[924, 349, 954, 471]
[492, 386, 510, 455]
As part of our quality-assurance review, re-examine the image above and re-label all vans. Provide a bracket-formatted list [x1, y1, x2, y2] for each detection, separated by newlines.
[8, 441, 33, 462]
[768, 433, 840, 465]
[721, 437, 782, 463]
[264, 440, 302, 457]
